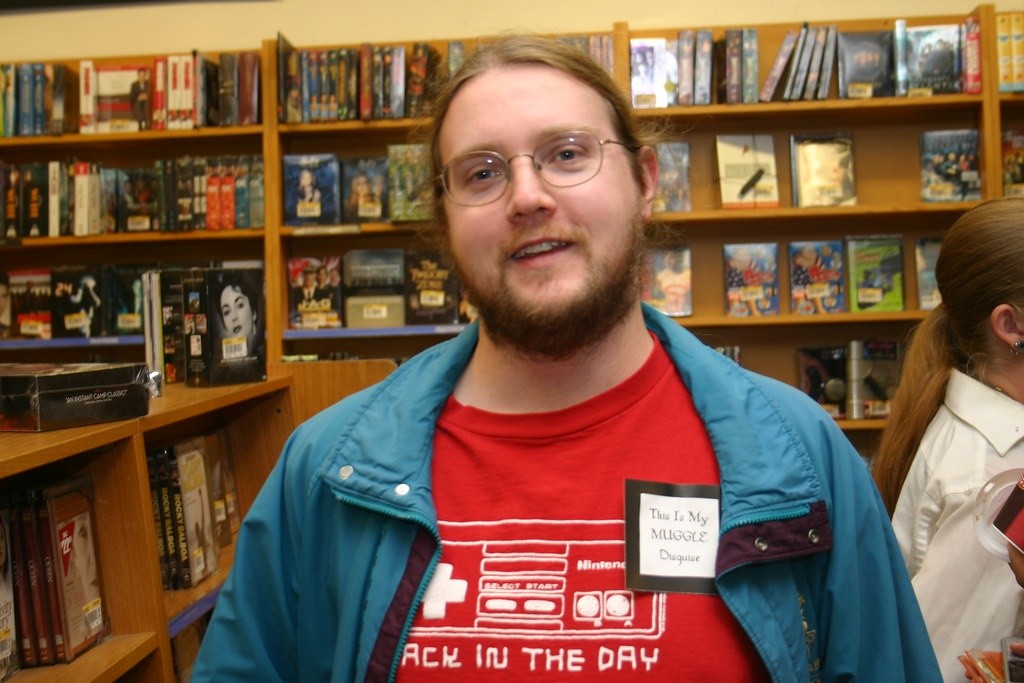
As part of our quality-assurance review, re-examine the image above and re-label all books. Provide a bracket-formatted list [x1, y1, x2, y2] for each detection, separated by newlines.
[567, 11, 1024, 109]
[921, 129, 1024, 204]
[1, 48, 267, 387]
[648, 127, 857, 214]
[707, 343, 742, 366]
[0, 423, 242, 683]
[992, 473, 1024, 555]
[797, 339, 908, 419]
[639, 233, 944, 318]
[274, 30, 481, 368]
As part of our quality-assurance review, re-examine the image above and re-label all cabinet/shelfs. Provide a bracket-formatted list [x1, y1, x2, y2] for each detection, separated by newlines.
[0, 50, 268, 348]
[276, 30, 613, 340]
[0, 375, 296, 683]
[629, 15, 983, 429]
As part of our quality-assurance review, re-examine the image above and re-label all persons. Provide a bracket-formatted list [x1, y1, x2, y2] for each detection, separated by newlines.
[214, 269, 265, 383]
[872, 198, 1024, 682]
[965, 541, 1023, 682]
[191, 36, 946, 682]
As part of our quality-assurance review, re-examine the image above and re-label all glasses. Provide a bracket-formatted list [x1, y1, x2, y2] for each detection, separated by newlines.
[433, 130, 642, 207]
